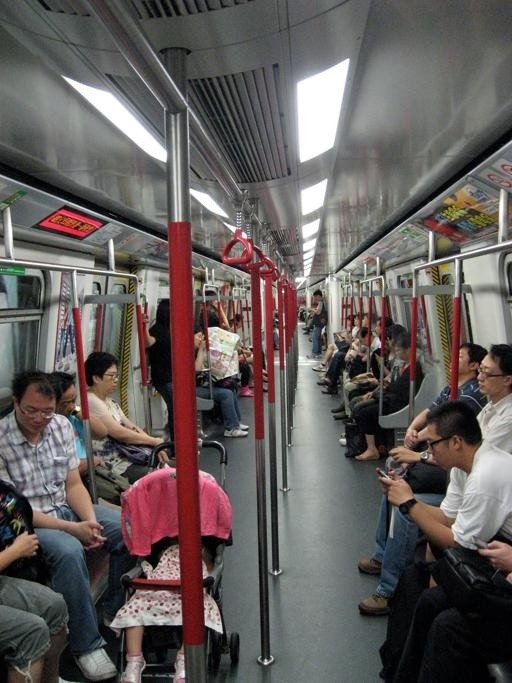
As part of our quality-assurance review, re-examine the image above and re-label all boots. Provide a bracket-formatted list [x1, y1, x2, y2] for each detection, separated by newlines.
[240, 385, 254, 397]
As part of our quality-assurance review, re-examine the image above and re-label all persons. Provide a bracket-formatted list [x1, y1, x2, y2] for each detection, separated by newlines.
[1, 289, 276, 683]
[304, 291, 326, 360]
[311, 314, 425, 458]
[357, 343, 511, 682]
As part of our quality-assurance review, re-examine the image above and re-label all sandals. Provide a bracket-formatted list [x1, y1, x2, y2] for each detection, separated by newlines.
[355, 449, 380, 460]
[173, 651, 185, 683]
[121, 653, 146, 683]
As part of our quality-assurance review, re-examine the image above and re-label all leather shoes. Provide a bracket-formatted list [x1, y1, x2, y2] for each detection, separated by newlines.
[331, 407, 348, 419]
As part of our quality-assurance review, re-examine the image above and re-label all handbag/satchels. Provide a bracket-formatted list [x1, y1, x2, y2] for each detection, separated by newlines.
[344, 415, 394, 458]
[107, 424, 158, 466]
[427, 548, 512, 618]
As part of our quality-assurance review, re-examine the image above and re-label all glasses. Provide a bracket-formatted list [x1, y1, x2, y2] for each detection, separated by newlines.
[16, 405, 54, 419]
[477, 368, 504, 377]
[426, 436, 455, 450]
[103, 373, 121, 380]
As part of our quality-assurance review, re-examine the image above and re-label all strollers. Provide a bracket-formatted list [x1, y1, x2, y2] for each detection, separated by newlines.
[112, 438, 240, 683]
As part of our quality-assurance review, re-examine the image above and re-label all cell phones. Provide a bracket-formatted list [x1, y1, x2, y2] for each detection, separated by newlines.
[375, 467, 390, 480]
[471, 535, 489, 549]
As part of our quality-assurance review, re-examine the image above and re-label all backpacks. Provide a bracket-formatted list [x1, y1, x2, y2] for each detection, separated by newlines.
[0, 479, 50, 585]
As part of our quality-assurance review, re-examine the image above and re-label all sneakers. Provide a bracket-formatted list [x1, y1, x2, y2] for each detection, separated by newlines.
[312, 363, 341, 394]
[223, 423, 249, 437]
[73, 647, 118, 681]
[358, 595, 390, 616]
[358, 559, 380, 574]
